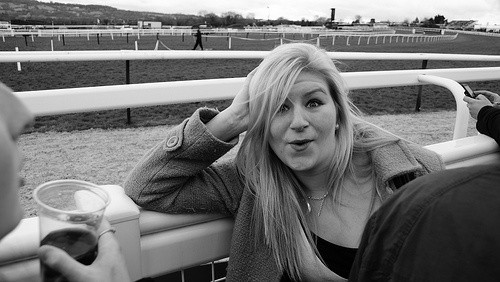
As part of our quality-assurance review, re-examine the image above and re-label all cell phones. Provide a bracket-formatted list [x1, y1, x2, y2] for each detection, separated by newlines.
[461, 84, 476, 98]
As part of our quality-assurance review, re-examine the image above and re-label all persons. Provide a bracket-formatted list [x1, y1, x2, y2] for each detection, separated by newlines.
[192, 30, 203, 50]
[0, 83, 129, 282]
[123, 43, 446, 282]
[463, 90, 500, 147]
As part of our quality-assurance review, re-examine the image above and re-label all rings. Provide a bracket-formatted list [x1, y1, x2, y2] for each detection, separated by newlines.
[97, 228, 116, 240]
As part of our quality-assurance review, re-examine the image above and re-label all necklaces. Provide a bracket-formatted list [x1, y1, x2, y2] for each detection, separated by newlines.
[308, 189, 330, 216]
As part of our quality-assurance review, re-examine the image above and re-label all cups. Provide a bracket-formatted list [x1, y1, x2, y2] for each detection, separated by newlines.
[33, 179, 112, 282]
